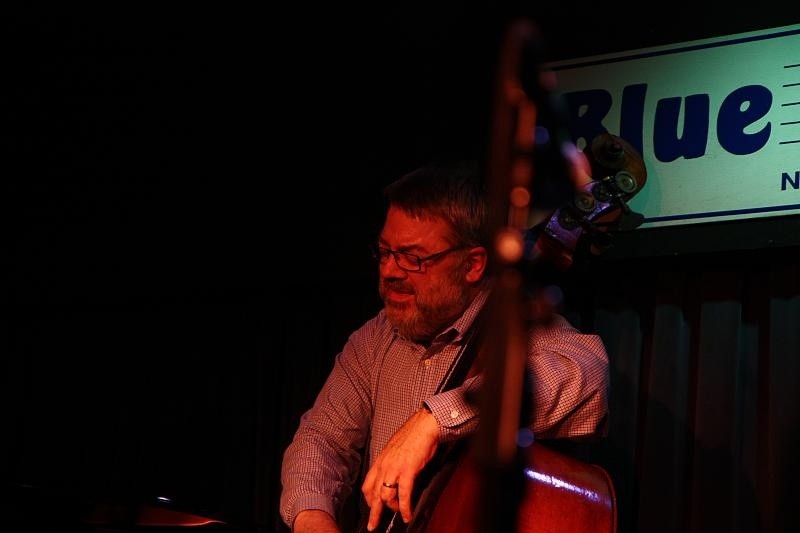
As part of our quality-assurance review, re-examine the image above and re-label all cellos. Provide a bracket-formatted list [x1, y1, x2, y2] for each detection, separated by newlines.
[318, 124, 648, 533]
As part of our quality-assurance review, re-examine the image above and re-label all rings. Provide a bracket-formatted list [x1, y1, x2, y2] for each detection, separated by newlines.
[383, 482, 398, 488]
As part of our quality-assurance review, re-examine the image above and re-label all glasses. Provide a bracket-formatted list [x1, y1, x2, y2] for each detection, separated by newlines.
[369, 243, 462, 271]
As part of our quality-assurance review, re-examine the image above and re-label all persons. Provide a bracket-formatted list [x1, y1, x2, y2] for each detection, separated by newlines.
[277, 166, 618, 533]
